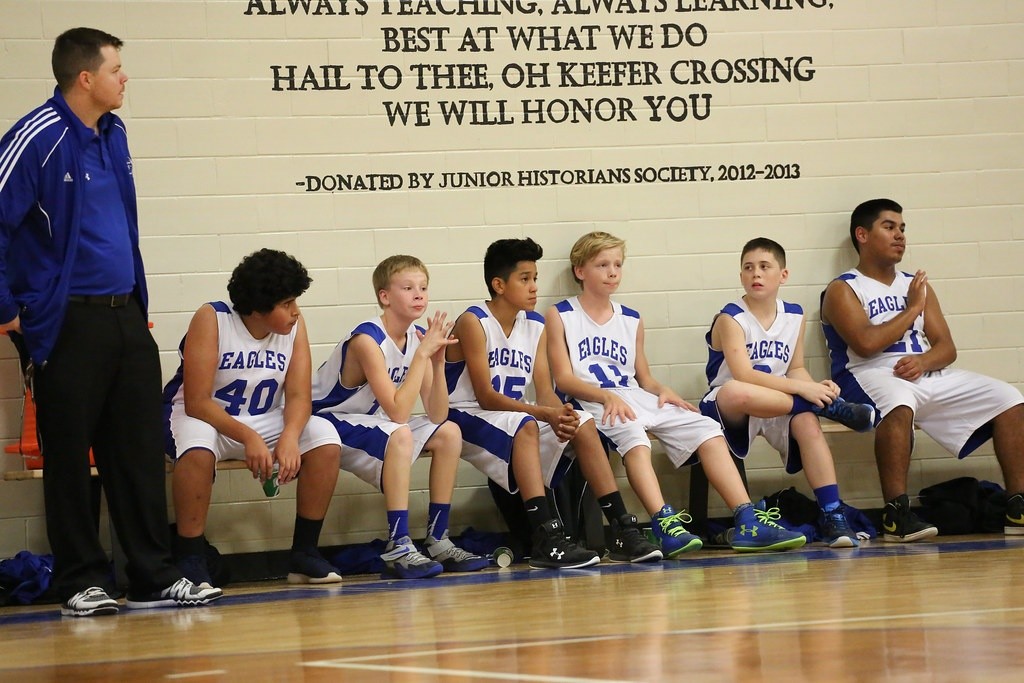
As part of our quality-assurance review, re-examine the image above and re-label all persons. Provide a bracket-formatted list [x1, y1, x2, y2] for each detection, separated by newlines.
[819, 198, 1024, 541]
[162, 248, 343, 590]
[0, 27, 223, 616]
[699, 238, 876, 547]
[545, 232, 807, 558]
[312, 254, 490, 579]
[445, 237, 663, 569]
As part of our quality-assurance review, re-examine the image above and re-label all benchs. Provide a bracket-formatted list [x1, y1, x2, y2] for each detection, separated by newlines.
[3, 416, 922, 593]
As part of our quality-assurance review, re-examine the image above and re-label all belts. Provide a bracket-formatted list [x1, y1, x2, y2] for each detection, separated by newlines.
[68, 291, 135, 308]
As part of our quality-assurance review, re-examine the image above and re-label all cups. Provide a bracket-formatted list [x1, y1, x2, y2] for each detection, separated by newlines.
[713, 526, 736, 544]
[258, 462, 281, 497]
[643, 528, 658, 546]
[492, 547, 514, 567]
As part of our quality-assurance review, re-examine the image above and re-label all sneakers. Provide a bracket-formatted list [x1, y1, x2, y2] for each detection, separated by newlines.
[882, 492, 939, 542]
[286, 549, 344, 583]
[603, 514, 664, 563]
[1002, 503, 1024, 535]
[60, 587, 120, 617]
[377, 535, 443, 580]
[821, 503, 860, 548]
[122, 576, 225, 610]
[819, 395, 876, 434]
[528, 518, 601, 569]
[420, 528, 490, 576]
[650, 504, 703, 559]
[184, 553, 215, 587]
[728, 504, 807, 555]
